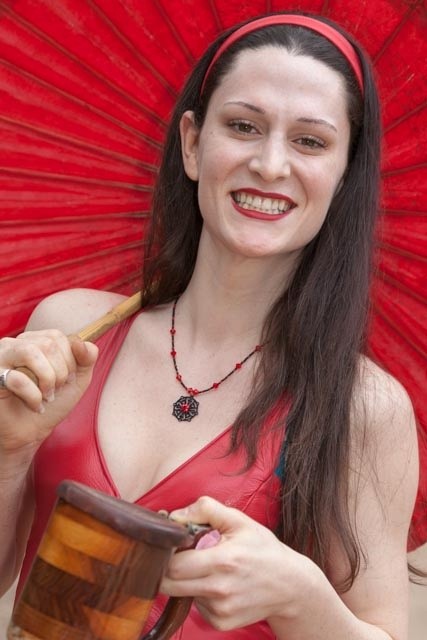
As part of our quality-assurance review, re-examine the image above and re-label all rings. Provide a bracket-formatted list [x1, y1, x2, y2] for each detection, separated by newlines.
[0, 368, 12, 389]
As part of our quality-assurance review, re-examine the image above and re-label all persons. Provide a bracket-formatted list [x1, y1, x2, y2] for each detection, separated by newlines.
[0, 9, 421, 640]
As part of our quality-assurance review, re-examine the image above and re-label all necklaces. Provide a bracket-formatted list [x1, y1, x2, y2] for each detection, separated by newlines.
[169, 296, 276, 423]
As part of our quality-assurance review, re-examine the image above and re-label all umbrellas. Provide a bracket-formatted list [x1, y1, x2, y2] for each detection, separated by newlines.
[0, 1, 427, 554]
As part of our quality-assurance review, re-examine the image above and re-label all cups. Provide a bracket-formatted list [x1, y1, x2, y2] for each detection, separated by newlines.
[7, 479, 205, 640]
